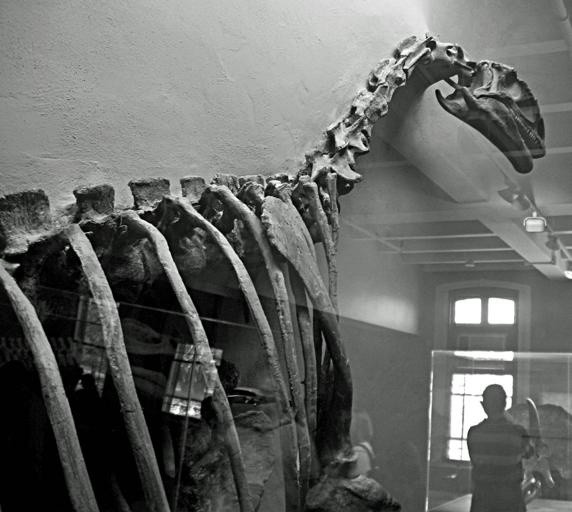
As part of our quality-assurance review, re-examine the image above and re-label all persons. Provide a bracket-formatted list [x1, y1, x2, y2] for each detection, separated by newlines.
[467, 384, 534, 512]
